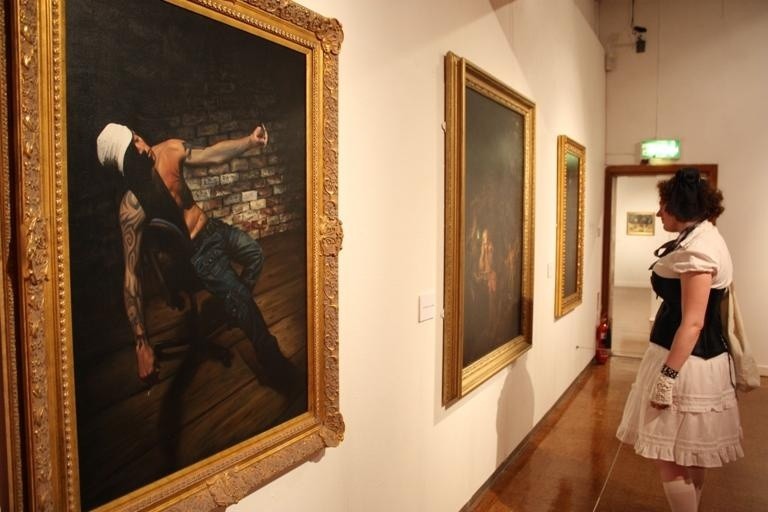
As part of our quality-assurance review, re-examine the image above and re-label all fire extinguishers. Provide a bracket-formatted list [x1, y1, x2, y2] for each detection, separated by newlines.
[595, 315, 612, 365]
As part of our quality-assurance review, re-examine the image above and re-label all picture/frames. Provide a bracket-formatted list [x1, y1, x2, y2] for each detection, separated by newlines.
[553, 132, 589, 321]
[439, 50, 538, 408]
[0, 1, 346, 511]
[626, 211, 656, 237]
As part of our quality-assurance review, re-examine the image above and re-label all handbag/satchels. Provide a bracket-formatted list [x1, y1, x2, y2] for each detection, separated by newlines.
[720, 282, 761, 392]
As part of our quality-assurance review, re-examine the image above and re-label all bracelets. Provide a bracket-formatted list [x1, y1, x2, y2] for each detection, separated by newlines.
[662, 364, 678, 379]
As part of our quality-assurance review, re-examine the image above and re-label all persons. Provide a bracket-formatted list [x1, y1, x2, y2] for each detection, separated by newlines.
[95, 121, 304, 387]
[617, 168, 744, 512]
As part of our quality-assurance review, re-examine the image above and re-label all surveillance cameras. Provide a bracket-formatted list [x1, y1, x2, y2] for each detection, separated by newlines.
[633, 26, 647, 32]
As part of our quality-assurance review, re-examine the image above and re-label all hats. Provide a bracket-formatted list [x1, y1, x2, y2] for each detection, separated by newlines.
[97, 123, 133, 176]
